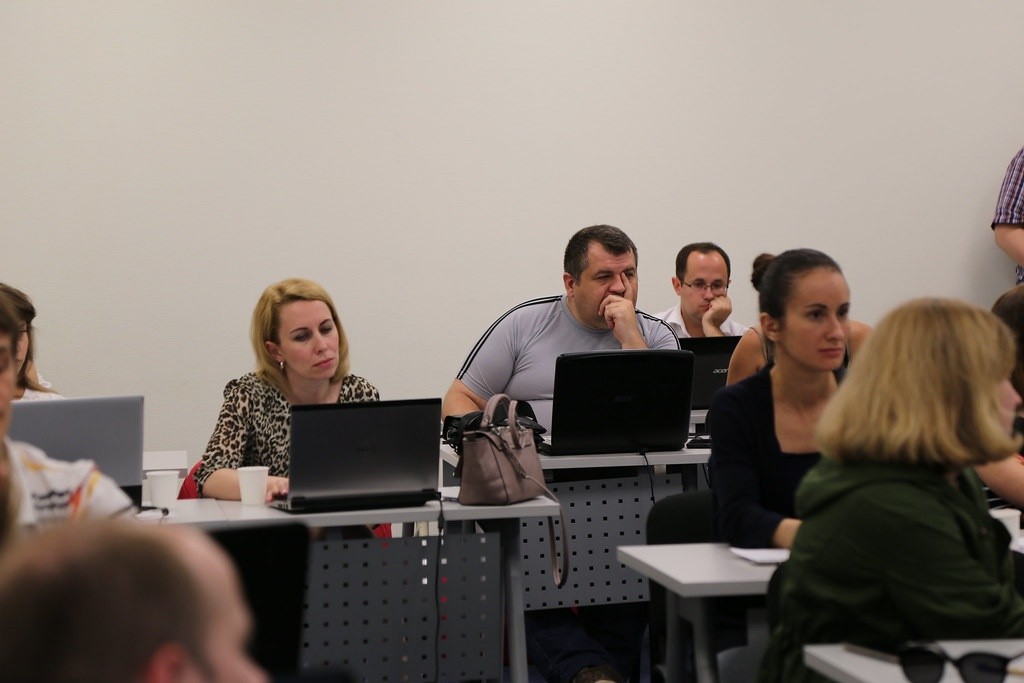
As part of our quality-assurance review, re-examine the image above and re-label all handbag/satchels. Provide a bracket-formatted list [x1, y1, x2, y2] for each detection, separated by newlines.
[457, 394, 546, 505]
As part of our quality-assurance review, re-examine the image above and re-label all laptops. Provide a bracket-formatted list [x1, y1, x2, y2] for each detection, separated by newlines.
[8, 394, 144, 515]
[539, 349, 696, 454]
[269, 398, 443, 511]
[678, 336, 741, 411]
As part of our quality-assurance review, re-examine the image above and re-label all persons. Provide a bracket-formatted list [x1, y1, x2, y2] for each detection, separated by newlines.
[709, 249, 850, 551]
[0, 516, 270, 683]
[0, 283, 137, 539]
[652, 242, 749, 338]
[991, 146, 1024, 285]
[440, 225, 682, 683]
[972, 286, 1024, 531]
[193, 277, 379, 541]
[755, 296, 1024, 683]
[726, 254, 875, 384]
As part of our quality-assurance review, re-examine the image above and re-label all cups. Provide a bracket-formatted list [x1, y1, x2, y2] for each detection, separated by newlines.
[989, 507, 1021, 546]
[145, 470, 179, 509]
[237, 466, 271, 505]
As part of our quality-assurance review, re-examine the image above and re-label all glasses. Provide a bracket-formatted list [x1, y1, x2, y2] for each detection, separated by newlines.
[901, 648, 1024, 683]
[680, 278, 731, 296]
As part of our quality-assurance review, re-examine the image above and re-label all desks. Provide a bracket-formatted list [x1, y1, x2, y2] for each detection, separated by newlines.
[138, 424, 1024, 683]
